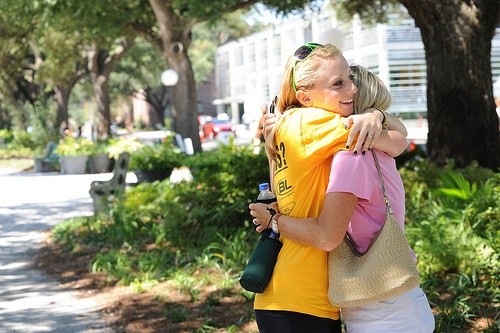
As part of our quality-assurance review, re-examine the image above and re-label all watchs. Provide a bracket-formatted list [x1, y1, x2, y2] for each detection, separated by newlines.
[272, 214, 281, 234]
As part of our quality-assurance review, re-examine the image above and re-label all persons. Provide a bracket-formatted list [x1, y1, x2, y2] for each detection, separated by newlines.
[248, 63, 436, 333]
[253, 42, 407, 333]
[255, 104, 269, 143]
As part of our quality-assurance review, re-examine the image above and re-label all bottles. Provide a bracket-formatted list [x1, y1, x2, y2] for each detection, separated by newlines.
[257, 183, 279, 239]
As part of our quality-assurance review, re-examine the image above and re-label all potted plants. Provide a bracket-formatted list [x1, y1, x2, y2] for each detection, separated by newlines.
[52, 133, 142, 175]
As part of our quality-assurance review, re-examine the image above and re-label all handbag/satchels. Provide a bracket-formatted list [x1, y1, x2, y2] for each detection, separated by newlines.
[328, 149, 420, 308]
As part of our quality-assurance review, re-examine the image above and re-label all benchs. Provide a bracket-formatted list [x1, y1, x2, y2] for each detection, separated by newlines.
[88, 151, 131, 215]
[32, 152, 58, 172]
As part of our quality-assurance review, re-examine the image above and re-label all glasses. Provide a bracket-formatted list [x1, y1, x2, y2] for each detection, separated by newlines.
[292, 42, 323, 91]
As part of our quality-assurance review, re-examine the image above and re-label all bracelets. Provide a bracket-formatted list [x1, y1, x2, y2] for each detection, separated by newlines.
[375, 107, 386, 124]
[266, 208, 278, 228]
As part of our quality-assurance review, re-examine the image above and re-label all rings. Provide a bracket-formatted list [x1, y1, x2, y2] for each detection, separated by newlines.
[368, 133, 373, 137]
